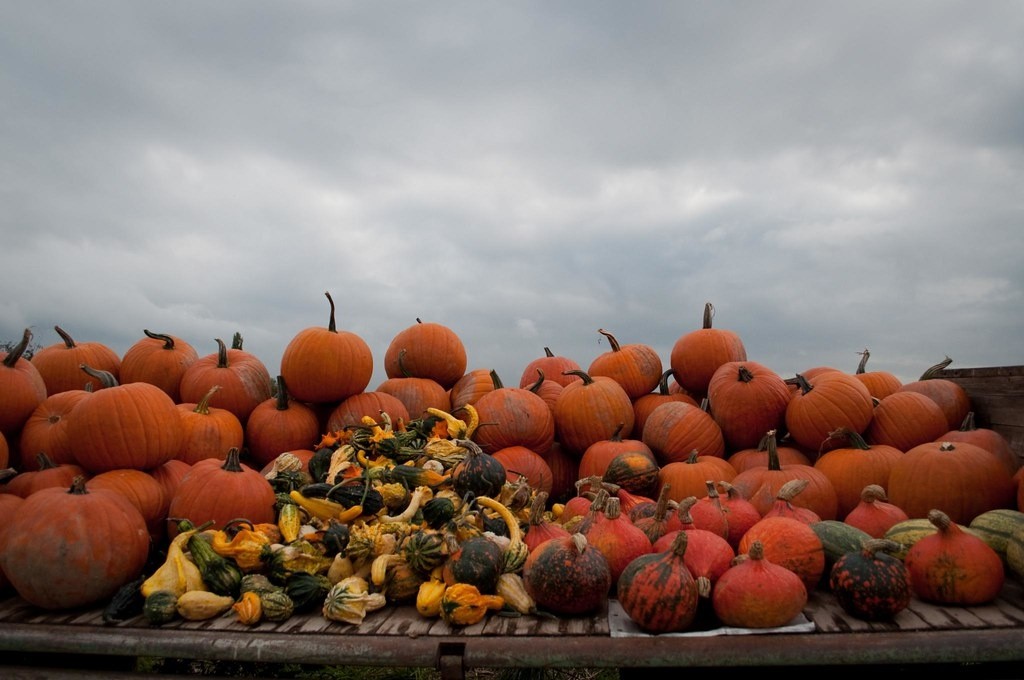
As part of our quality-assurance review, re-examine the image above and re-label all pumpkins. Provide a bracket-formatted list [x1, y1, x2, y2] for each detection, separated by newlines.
[0, 289, 1024, 635]
